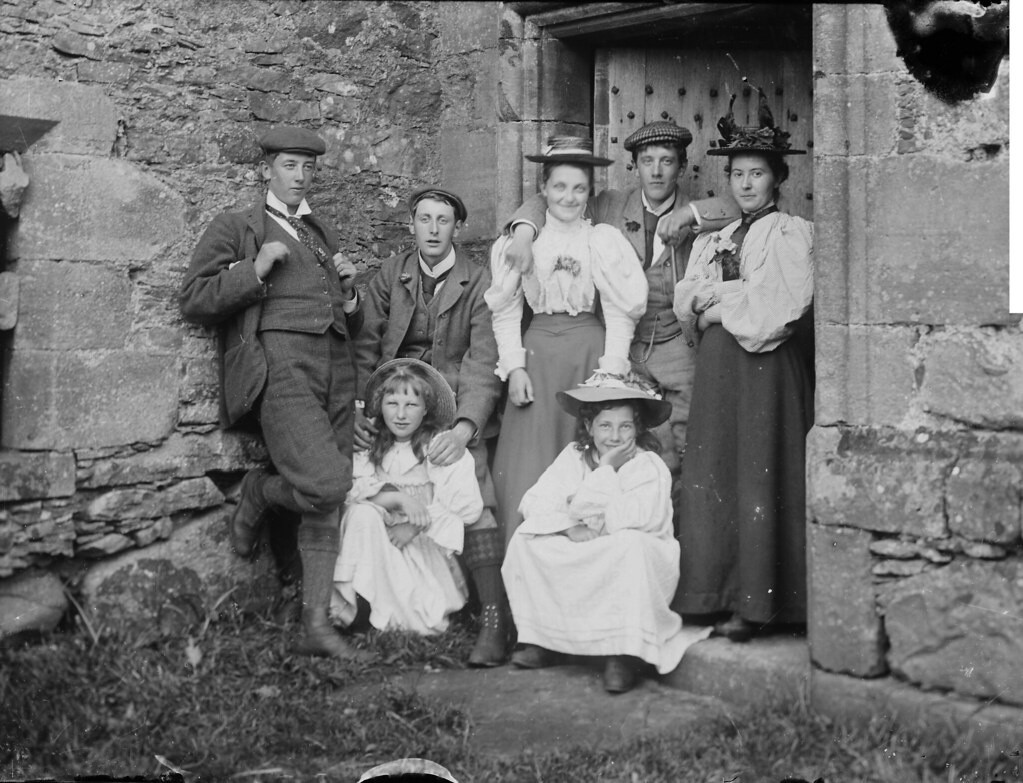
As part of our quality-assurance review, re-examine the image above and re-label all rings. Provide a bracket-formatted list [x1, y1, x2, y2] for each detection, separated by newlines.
[627, 453, 635, 459]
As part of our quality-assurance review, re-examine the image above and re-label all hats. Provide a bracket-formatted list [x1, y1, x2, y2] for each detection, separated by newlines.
[624, 121, 693, 152]
[524, 135, 616, 166]
[260, 126, 327, 156]
[409, 184, 468, 224]
[707, 127, 807, 155]
[556, 372, 672, 430]
[363, 357, 456, 432]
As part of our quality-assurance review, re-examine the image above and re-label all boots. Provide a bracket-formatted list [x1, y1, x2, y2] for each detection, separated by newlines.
[463, 526, 510, 666]
[290, 607, 382, 664]
[230, 469, 275, 556]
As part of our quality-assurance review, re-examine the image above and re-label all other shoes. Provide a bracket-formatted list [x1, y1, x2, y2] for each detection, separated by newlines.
[511, 644, 589, 669]
[601, 655, 636, 692]
[716, 613, 779, 643]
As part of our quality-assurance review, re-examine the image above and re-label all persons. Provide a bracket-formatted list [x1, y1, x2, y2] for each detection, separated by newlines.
[328, 357, 485, 638]
[482, 135, 649, 558]
[176, 126, 379, 665]
[499, 379, 681, 693]
[352, 188, 504, 668]
[502, 120, 743, 462]
[669, 127, 816, 644]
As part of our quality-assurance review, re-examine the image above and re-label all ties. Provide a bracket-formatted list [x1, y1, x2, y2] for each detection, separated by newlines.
[721, 205, 780, 281]
[642, 200, 676, 270]
[265, 203, 329, 265]
[417, 260, 454, 306]
